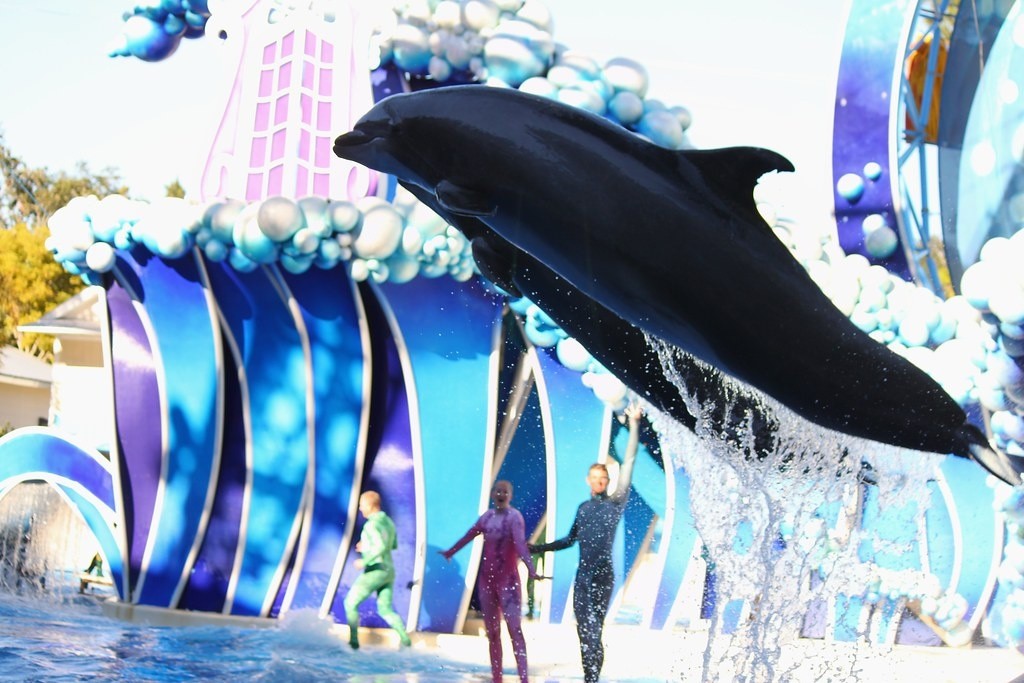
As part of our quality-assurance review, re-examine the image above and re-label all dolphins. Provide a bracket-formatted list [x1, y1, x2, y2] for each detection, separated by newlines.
[329, 85, 1020, 493]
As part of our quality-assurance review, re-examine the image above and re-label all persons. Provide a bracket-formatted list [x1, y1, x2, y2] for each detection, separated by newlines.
[343, 490, 412, 651]
[517, 402, 643, 683]
[436, 479, 554, 683]
